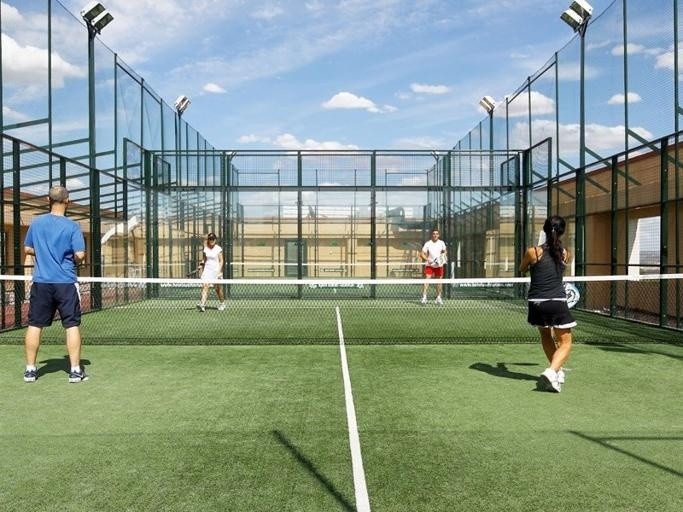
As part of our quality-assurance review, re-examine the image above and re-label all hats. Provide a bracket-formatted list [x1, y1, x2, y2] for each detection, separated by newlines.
[208, 233, 216, 240]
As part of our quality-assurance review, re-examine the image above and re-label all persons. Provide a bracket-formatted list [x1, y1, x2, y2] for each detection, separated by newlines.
[197, 233, 226, 312]
[23, 185, 91, 383]
[420, 229, 448, 304]
[518, 215, 578, 393]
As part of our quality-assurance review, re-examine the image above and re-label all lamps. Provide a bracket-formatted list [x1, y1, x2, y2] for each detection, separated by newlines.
[80, 0, 105, 22]
[569, 0, 593, 20]
[559, 8, 583, 30]
[179, 99, 191, 113]
[482, 96, 496, 108]
[175, 95, 187, 109]
[91, 10, 114, 31]
[479, 99, 491, 112]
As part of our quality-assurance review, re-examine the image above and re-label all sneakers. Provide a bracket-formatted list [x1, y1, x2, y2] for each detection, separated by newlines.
[422, 297, 427, 304]
[435, 298, 444, 305]
[24, 366, 40, 382]
[196, 303, 206, 312]
[218, 304, 226, 311]
[68, 368, 89, 383]
[541, 368, 565, 393]
